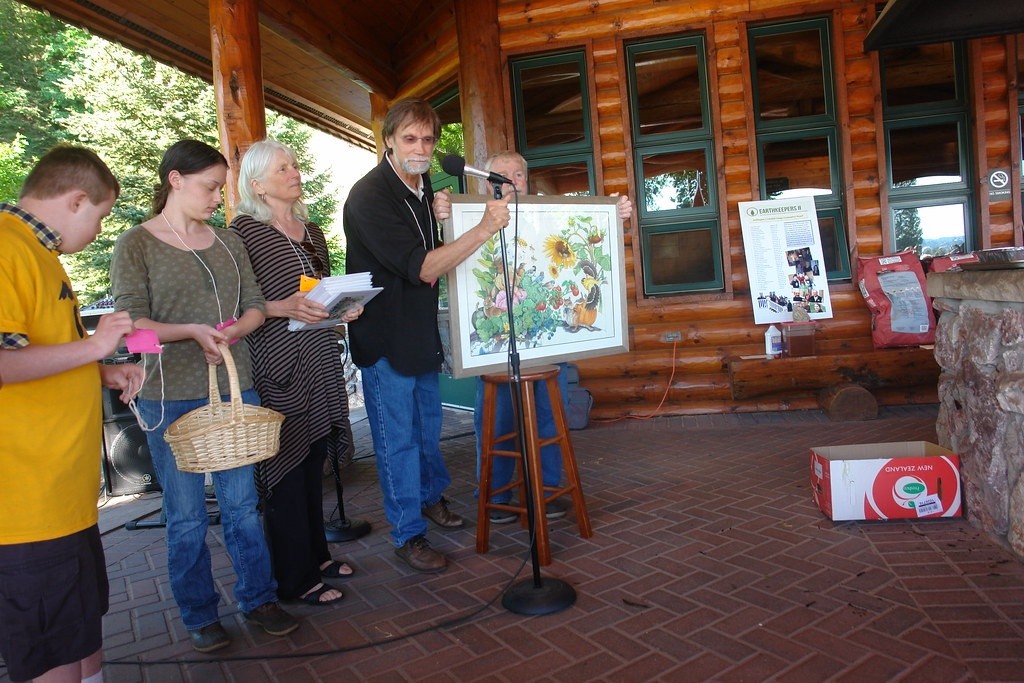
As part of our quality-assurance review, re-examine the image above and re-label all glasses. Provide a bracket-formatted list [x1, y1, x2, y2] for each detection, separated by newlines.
[299, 240, 323, 273]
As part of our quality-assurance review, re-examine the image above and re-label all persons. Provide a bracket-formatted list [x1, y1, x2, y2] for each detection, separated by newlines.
[109, 138, 301, 651]
[804, 290, 823, 302]
[794, 289, 803, 301]
[430, 150, 631, 522]
[768, 291, 792, 312]
[797, 301, 811, 313]
[813, 304, 823, 313]
[0, 146, 145, 683]
[788, 248, 818, 288]
[343, 95, 514, 576]
[228, 138, 367, 607]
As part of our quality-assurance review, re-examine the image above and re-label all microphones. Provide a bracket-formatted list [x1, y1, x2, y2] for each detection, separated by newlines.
[442, 154, 513, 185]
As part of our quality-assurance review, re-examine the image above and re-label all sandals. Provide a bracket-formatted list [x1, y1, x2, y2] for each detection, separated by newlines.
[300, 582, 345, 603]
[320, 560, 353, 577]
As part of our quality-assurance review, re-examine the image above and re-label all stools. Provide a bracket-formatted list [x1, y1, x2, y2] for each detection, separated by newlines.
[476, 368, 592, 565]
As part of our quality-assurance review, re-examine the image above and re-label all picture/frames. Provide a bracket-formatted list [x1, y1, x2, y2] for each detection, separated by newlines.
[443, 193, 628, 379]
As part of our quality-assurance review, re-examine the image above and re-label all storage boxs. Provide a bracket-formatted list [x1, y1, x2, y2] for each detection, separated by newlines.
[809, 440, 962, 521]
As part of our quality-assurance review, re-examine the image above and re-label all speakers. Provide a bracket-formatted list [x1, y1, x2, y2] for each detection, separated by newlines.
[96, 347, 162, 497]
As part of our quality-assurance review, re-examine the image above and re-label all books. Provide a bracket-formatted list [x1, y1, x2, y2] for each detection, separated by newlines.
[288, 272, 383, 333]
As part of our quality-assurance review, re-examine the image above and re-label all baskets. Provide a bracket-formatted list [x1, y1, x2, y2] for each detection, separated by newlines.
[163, 342, 285, 474]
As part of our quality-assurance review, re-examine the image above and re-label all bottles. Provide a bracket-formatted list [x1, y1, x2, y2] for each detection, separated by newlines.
[764, 323, 783, 355]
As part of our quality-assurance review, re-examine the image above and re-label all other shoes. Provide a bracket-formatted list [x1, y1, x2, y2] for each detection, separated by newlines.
[491, 508, 516, 522]
[546, 502, 567, 517]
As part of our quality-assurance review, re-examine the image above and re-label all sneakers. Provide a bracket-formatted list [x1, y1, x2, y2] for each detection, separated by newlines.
[395, 535, 446, 573]
[189, 621, 230, 653]
[424, 497, 464, 530]
[244, 602, 299, 636]
